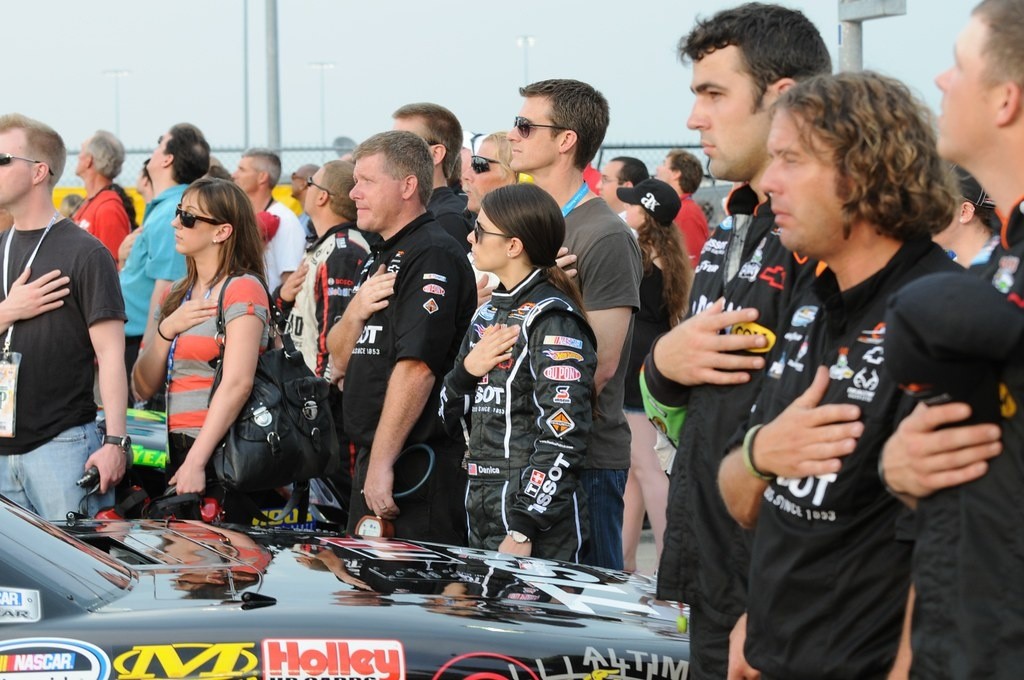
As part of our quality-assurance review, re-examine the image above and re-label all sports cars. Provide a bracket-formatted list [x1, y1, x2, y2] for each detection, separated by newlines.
[1, 485, 694, 680]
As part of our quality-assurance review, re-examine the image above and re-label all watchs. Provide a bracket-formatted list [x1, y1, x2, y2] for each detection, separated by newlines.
[507, 530, 531, 544]
[101, 433, 132, 453]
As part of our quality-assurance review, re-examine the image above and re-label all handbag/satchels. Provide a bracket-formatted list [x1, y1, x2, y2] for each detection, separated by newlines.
[206, 270, 342, 492]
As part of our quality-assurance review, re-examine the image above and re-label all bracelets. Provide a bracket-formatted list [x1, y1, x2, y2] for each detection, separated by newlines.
[742, 423, 779, 481]
[158, 320, 175, 342]
[878, 450, 912, 498]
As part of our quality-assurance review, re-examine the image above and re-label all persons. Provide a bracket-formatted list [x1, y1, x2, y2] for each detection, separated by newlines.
[59, 518, 630, 625]
[714, 71, 969, 679]
[876, 0, 1024, 680]
[0, 78, 713, 576]
[640, 2, 832, 680]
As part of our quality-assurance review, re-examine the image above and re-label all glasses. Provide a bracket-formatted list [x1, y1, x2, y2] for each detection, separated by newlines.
[471, 155, 500, 174]
[0, 153, 55, 177]
[292, 172, 304, 181]
[175, 203, 223, 228]
[474, 219, 513, 243]
[514, 116, 580, 140]
[306, 176, 330, 196]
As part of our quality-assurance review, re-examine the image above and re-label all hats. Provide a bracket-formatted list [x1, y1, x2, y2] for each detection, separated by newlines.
[616, 178, 682, 227]
[881, 270, 1023, 427]
